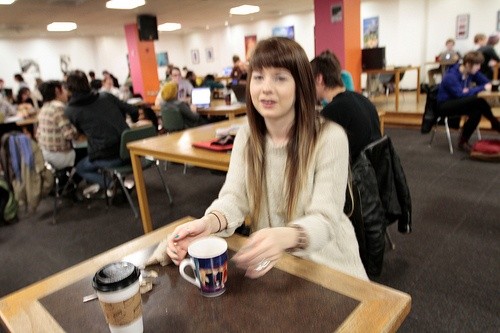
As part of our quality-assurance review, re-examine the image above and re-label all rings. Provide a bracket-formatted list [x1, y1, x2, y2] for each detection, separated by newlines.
[258, 258, 270, 268]
[174, 242, 178, 246]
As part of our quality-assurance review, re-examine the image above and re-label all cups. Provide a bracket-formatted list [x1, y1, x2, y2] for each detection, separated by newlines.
[179, 236, 228, 298]
[91, 261, 144, 333]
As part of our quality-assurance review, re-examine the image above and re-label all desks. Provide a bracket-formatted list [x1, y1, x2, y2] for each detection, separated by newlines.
[191, 98, 247, 119]
[124, 115, 250, 232]
[366, 67, 422, 113]
[0, 217, 412, 333]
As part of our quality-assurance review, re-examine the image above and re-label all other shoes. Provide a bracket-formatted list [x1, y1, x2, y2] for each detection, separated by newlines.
[82, 182, 100, 198]
[459, 137, 472, 152]
[106, 178, 117, 198]
[492, 121, 500, 131]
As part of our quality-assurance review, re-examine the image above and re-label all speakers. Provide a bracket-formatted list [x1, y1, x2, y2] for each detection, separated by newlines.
[138, 14, 158, 41]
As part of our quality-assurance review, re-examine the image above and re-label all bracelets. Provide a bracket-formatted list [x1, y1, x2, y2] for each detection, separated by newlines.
[208, 212, 221, 233]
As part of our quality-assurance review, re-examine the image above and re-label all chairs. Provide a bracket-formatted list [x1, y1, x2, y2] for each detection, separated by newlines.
[104, 125, 173, 221]
[159, 104, 199, 175]
[426, 112, 482, 153]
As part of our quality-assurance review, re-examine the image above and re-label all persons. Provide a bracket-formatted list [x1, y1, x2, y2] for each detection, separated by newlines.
[428, 33, 500, 153]
[0, 50, 382, 204]
[166, 37, 369, 280]
